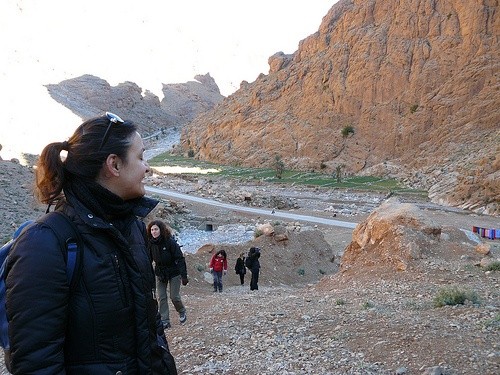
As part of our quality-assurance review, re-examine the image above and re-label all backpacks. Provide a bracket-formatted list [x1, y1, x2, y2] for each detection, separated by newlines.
[0, 209, 83, 349]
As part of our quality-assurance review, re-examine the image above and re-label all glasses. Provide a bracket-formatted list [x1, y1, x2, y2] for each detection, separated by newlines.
[96, 111, 124, 154]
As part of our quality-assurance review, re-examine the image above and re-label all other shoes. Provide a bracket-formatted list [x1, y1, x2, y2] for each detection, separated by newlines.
[219, 289, 224, 292]
[213, 287, 217, 292]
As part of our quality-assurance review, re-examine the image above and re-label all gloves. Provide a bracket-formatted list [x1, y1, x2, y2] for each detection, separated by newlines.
[181, 271, 189, 285]
[224, 270, 227, 275]
[210, 268, 213, 274]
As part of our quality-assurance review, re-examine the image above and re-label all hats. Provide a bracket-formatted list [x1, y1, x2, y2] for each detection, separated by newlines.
[239, 252, 245, 257]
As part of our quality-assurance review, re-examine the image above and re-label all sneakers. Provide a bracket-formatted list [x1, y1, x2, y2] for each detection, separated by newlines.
[179, 309, 187, 322]
[163, 319, 170, 329]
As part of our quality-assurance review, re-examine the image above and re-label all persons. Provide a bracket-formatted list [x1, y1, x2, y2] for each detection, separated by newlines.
[209, 249, 227, 293]
[0, 220, 36, 373]
[235, 252, 247, 286]
[4, 115, 175, 375]
[247, 246, 261, 291]
[144, 220, 189, 330]
